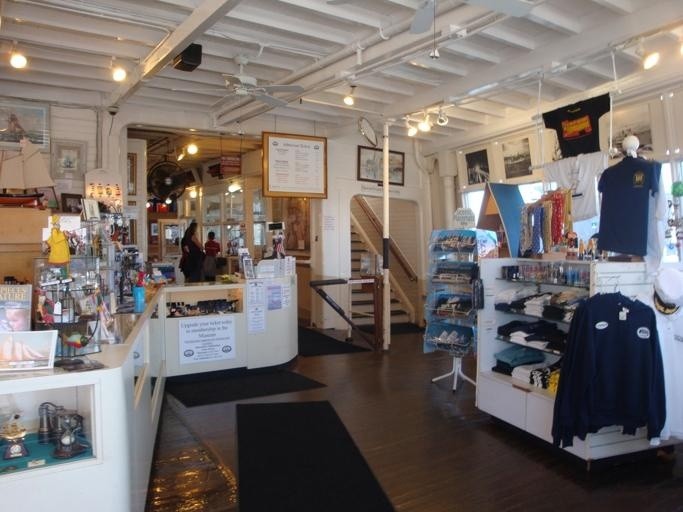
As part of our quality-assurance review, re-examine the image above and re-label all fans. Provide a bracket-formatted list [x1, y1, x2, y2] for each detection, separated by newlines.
[145, 159, 191, 203]
[173, 53, 304, 110]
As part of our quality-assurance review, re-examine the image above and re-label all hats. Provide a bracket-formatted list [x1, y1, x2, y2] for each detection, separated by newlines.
[654, 268, 683, 315]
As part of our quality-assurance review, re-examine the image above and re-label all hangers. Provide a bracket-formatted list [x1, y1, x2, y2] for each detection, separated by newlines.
[419, 205, 500, 397]
[597, 134, 662, 257]
[607, 274, 624, 298]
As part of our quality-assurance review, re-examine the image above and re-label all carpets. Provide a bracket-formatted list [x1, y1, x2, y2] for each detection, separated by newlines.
[296, 323, 376, 359]
[163, 362, 331, 410]
[234, 398, 392, 512]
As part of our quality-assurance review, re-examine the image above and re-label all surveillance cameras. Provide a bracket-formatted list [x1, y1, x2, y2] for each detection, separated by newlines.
[106, 105, 120, 118]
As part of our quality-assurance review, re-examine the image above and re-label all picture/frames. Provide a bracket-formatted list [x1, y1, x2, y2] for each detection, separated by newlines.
[355, 145, 406, 187]
[0, 94, 52, 155]
[126, 152, 138, 196]
[260, 131, 329, 200]
[48, 137, 88, 183]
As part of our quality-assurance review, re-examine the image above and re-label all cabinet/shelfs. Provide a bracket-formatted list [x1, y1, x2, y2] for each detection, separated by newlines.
[474, 250, 683, 473]
[0, 272, 165, 512]
[157, 271, 303, 388]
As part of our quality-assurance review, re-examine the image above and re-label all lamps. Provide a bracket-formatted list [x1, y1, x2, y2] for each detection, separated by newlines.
[416, 111, 432, 133]
[435, 107, 449, 127]
[634, 42, 661, 72]
[342, 84, 356, 108]
[402, 116, 420, 139]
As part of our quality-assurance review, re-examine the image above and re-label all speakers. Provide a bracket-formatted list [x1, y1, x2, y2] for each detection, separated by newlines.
[174, 43, 202, 72]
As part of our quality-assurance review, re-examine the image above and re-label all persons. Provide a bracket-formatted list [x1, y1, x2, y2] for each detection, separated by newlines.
[179, 227, 190, 283]
[1, 302, 28, 332]
[188, 220, 206, 281]
[205, 231, 221, 257]
